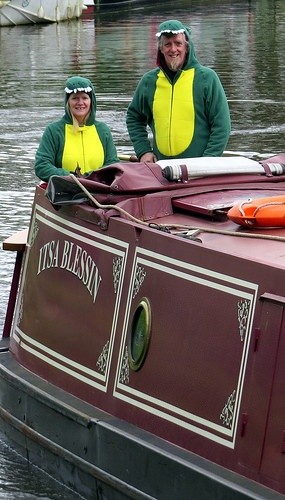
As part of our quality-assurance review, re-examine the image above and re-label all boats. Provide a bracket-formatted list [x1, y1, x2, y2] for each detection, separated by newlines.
[0, 153, 285, 499]
[1, 0, 93, 27]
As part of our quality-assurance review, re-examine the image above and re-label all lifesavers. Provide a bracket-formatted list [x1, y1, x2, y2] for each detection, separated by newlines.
[227, 194, 285, 227]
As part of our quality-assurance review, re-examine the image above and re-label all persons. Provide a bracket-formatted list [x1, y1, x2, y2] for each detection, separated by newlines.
[34, 76, 120, 183]
[125, 19, 231, 163]
